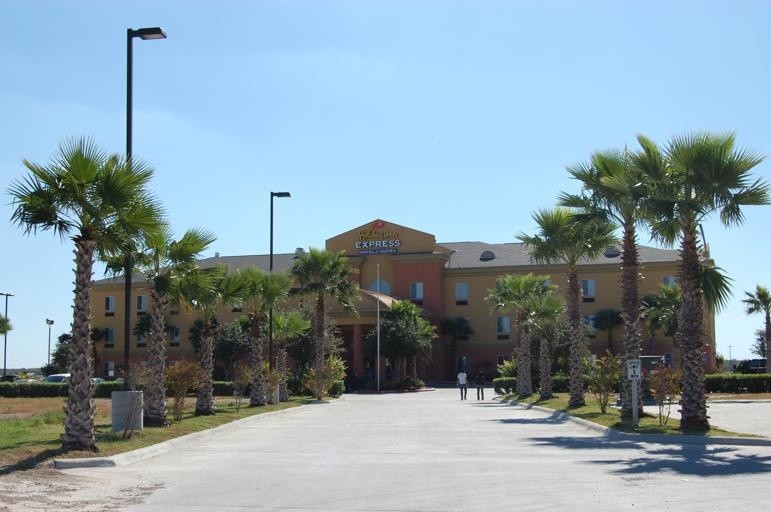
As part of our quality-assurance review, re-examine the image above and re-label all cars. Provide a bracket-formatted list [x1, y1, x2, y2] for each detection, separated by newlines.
[733, 358, 768, 374]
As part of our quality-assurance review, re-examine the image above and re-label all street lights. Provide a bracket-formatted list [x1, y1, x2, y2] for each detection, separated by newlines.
[265, 190, 291, 372]
[45, 318, 54, 366]
[0, 292, 15, 376]
[117, 25, 168, 391]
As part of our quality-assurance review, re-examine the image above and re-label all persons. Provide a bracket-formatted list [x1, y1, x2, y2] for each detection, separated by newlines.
[474, 370, 488, 400]
[456, 367, 468, 400]
